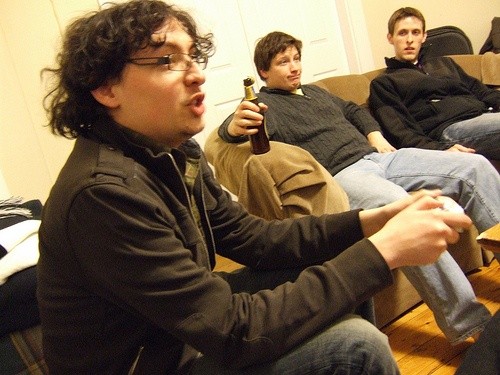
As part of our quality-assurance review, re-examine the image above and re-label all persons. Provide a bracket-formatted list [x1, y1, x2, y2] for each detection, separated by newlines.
[217, 30, 500, 348]
[36, 0, 472, 375]
[368, 8, 500, 162]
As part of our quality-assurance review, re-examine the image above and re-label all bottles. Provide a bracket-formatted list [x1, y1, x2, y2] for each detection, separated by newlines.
[243, 77, 271, 155]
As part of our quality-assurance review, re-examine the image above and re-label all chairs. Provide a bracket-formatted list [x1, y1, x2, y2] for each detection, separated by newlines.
[420, 26, 474, 57]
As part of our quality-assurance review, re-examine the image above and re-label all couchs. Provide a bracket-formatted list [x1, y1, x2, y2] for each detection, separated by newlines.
[204, 52, 500, 330]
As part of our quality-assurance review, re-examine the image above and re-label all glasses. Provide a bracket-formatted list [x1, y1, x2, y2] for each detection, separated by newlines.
[126, 54, 208, 71]
